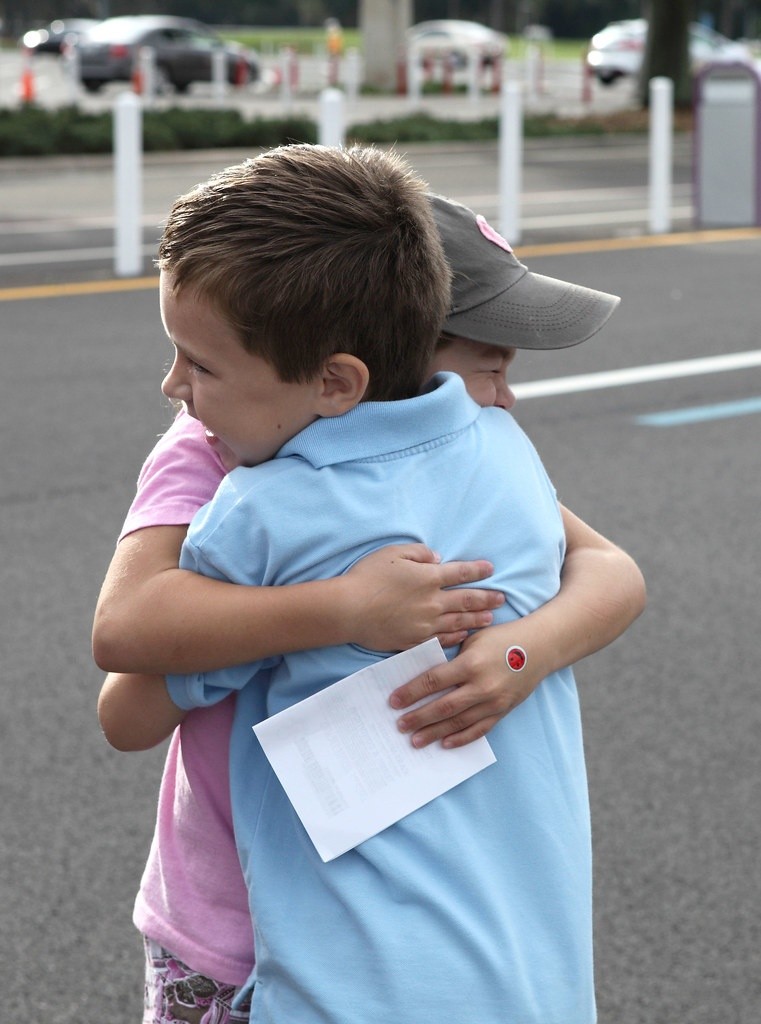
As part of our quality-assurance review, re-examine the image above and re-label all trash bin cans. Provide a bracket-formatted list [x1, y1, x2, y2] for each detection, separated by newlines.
[690, 54, 761, 228]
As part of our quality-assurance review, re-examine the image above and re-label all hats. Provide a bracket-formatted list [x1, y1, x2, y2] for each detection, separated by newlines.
[421, 193, 623, 350]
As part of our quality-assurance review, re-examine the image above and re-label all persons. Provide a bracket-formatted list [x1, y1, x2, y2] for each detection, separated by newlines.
[96, 189, 650, 1024]
[95, 138, 595, 1024]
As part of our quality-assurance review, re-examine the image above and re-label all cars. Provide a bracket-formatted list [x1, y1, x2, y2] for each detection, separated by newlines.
[355, 16, 515, 92]
[60, 12, 262, 98]
[584, 15, 761, 107]
[17, 16, 102, 56]
